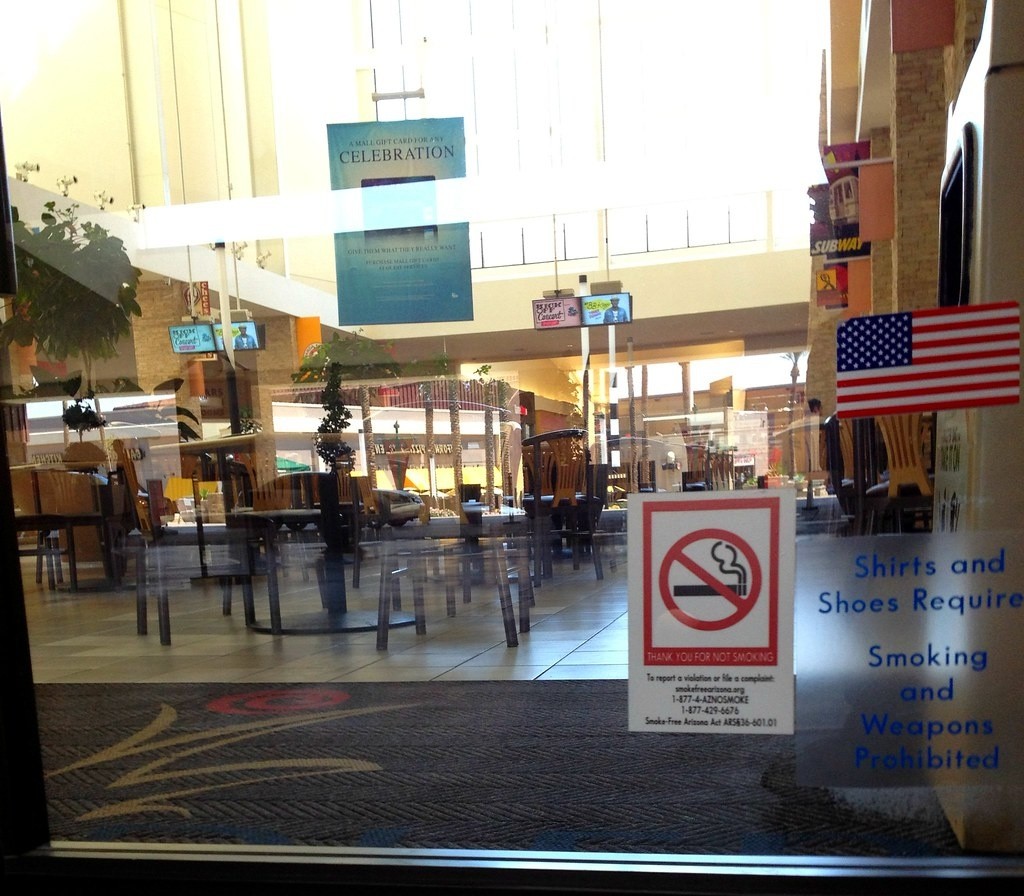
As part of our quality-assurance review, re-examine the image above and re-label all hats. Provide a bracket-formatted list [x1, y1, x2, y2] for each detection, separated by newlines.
[610, 296, 619, 301]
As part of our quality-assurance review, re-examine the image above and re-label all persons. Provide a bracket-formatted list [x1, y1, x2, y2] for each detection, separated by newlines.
[235, 325, 257, 349]
[809, 399, 823, 416]
[603, 296, 629, 323]
[112, 439, 138, 495]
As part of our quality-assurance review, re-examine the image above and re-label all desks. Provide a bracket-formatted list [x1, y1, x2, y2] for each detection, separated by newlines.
[148, 434, 420, 634]
[9, 460, 127, 591]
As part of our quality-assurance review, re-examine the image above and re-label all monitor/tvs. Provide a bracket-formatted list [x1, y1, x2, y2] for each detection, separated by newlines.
[169, 321, 261, 354]
[532, 292, 632, 330]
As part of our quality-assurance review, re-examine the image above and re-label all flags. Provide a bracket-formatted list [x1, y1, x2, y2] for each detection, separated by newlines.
[837, 298, 1021, 420]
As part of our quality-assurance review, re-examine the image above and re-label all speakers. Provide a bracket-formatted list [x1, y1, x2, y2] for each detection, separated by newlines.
[219, 309, 251, 322]
[590, 280, 622, 295]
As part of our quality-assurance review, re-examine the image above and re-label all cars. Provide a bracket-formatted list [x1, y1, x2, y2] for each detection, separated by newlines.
[235, 472, 424, 535]
[589, 438, 753, 491]
[861, 464, 1008, 529]
[27, 472, 175, 535]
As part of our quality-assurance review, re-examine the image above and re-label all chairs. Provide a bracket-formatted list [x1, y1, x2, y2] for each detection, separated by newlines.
[821, 406, 936, 536]
[6, 429, 606, 652]
